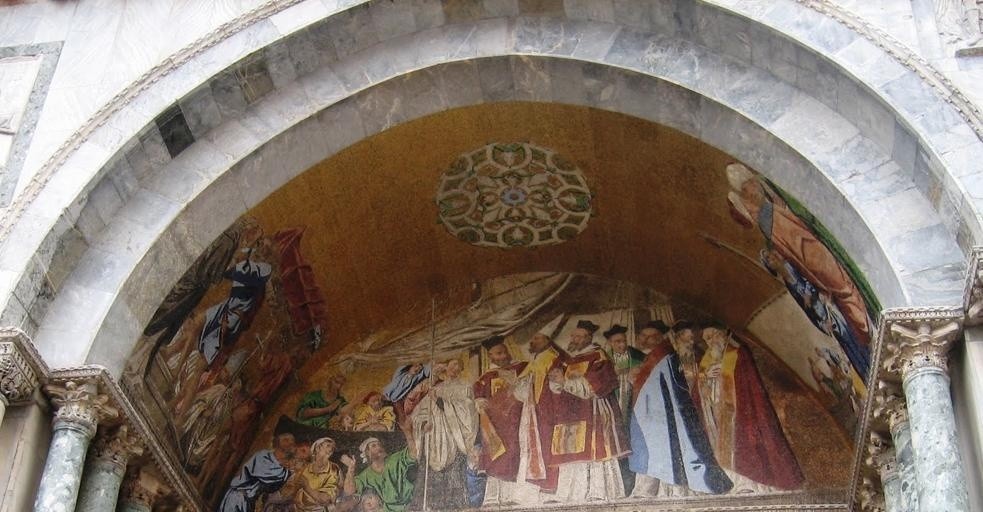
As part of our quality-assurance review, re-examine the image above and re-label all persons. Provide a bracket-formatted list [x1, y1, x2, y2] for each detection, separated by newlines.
[158, 238, 273, 431]
[188, 373, 250, 452]
[726, 162, 871, 350]
[194, 343, 293, 508]
[757, 245, 871, 385]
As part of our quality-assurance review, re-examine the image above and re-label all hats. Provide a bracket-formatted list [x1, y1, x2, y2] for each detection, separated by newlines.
[362, 391, 381, 405]
[480, 312, 727, 352]
